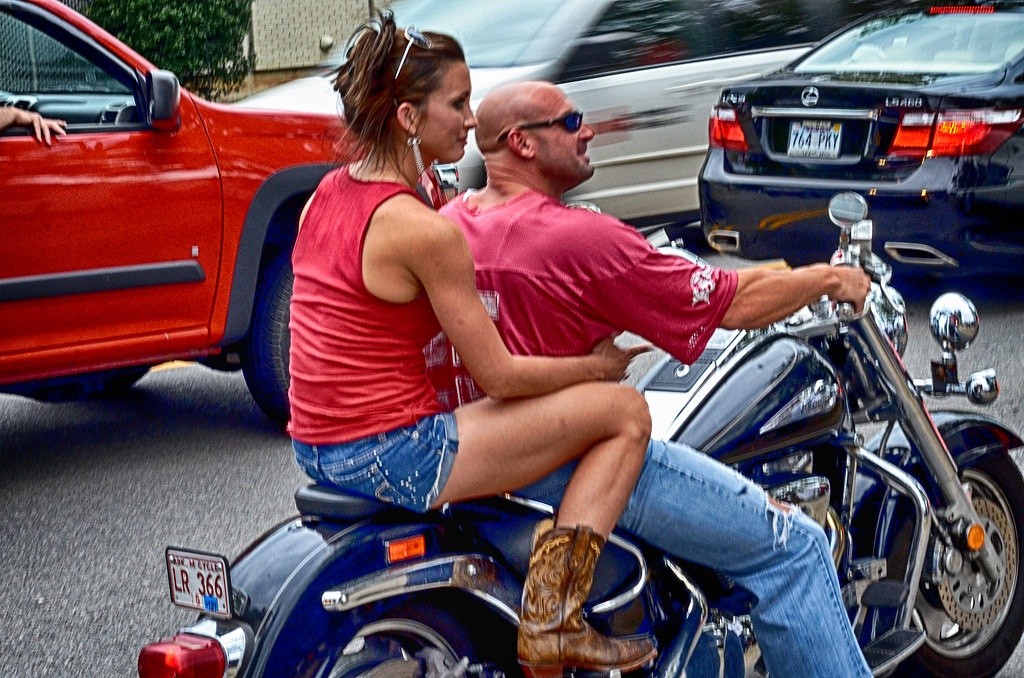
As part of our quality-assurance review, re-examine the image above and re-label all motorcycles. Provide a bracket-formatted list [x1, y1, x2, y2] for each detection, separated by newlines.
[137, 192, 1024, 678]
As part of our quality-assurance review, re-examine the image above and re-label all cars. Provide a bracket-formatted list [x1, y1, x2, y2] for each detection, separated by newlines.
[215, 0, 900, 254]
[697, 0, 1024, 302]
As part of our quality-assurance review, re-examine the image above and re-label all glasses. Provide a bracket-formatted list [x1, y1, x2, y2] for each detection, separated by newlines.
[391, 25, 432, 111]
[496, 109, 583, 144]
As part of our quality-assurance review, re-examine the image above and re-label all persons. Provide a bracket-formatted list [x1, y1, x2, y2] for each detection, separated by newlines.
[286, 11, 659, 678]
[0, 107, 68, 145]
[424, 79, 875, 678]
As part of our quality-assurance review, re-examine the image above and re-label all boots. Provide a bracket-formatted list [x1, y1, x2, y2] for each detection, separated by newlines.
[518, 517, 658, 678]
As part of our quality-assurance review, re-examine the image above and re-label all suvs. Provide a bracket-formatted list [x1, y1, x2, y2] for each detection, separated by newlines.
[0, 0, 452, 436]
